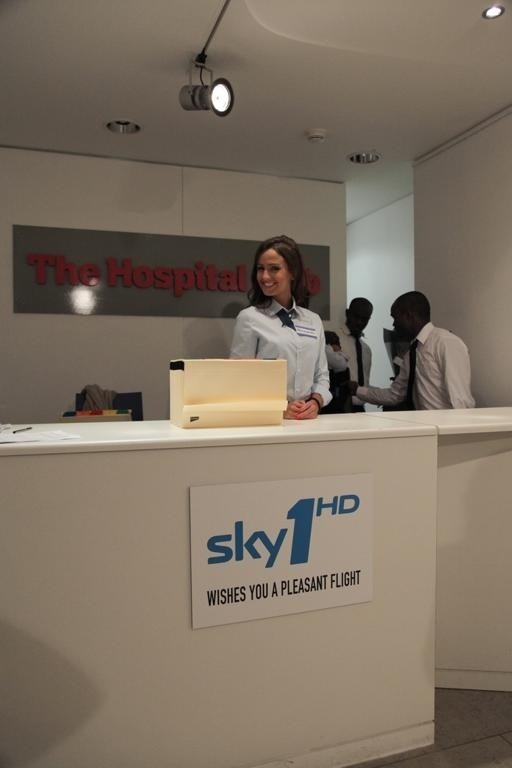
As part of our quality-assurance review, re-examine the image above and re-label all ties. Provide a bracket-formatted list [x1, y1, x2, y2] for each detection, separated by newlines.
[406, 339, 418, 410]
[350, 331, 364, 386]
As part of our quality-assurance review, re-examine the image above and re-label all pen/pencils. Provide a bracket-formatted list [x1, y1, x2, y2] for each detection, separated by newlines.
[13, 426, 33, 434]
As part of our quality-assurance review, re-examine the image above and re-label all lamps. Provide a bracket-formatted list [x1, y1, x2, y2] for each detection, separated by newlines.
[180, 57, 238, 118]
